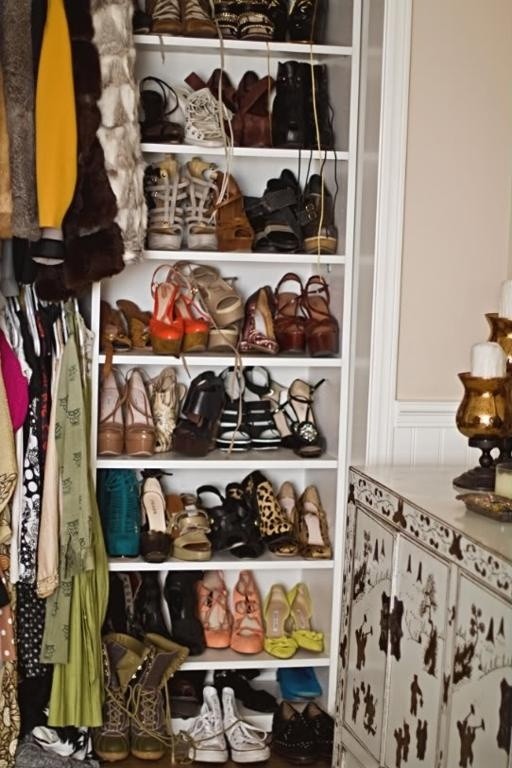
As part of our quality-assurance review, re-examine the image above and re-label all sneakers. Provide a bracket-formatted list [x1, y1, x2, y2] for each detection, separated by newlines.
[171, 668, 334, 765]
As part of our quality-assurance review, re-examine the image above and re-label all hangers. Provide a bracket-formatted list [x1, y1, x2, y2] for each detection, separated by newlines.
[0, 281, 84, 360]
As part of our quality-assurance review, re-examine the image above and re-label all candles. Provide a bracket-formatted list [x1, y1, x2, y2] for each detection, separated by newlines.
[469, 340, 507, 380]
[498, 278, 512, 321]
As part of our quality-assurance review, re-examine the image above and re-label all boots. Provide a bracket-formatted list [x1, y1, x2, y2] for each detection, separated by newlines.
[100, 631, 189, 761]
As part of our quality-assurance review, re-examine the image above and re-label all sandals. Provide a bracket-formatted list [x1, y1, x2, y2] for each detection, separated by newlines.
[145, 159, 338, 255]
[133, 0, 321, 43]
[141, 57, 335, 149]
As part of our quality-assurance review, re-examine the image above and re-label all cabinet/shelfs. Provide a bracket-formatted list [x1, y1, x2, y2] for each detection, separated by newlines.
[330, 464, 512, 768]
[87, 0, 362, 738]
